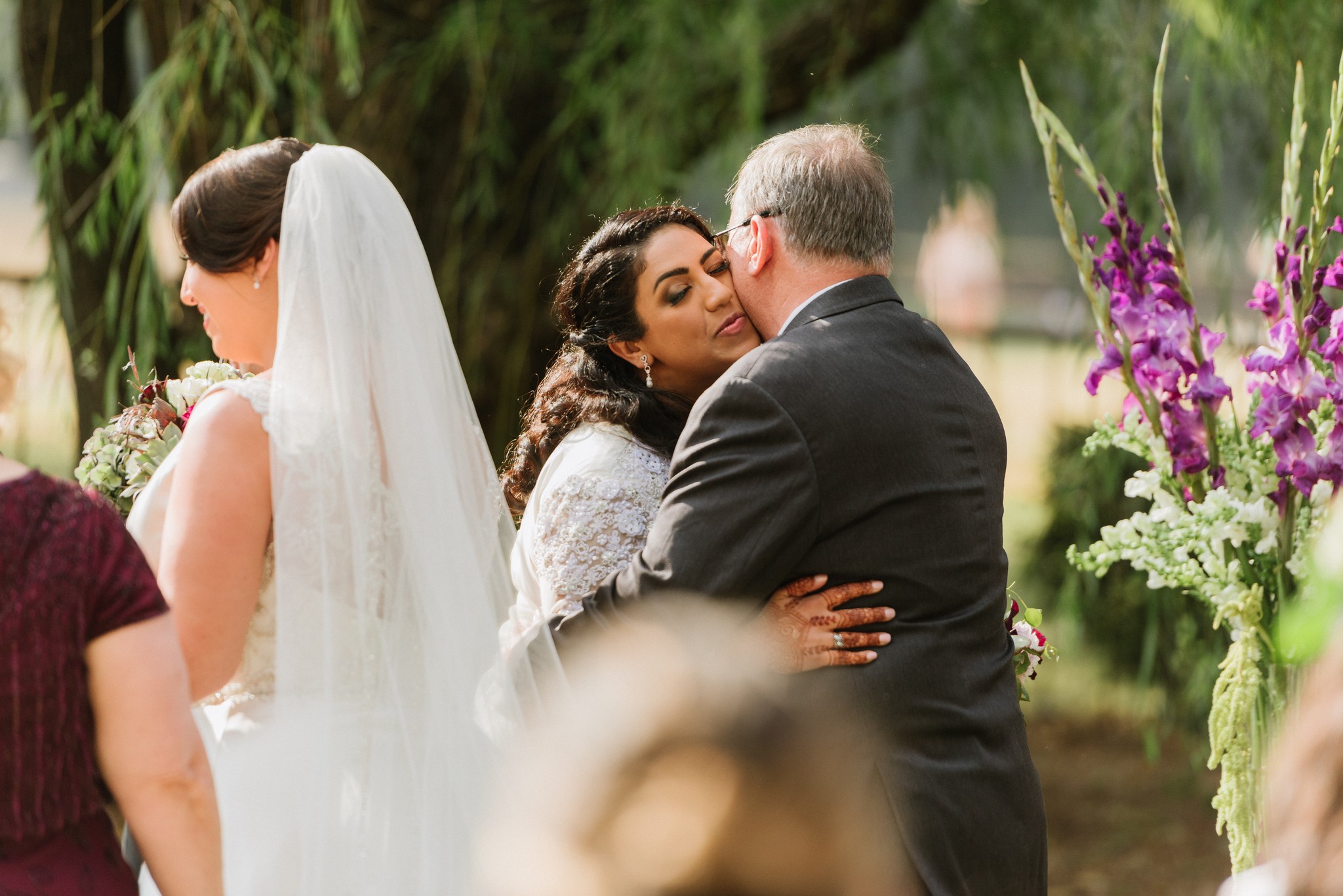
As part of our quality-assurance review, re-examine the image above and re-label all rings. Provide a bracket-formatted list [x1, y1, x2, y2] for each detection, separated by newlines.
[834, 630, 845, 648]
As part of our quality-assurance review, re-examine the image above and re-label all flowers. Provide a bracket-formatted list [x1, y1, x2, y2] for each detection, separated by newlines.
[72, 341, 256, 519]
[1016, 19, 1343, 884]
[1001, 578, 1061, 704]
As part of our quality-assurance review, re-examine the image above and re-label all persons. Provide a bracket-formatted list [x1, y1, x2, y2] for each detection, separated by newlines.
[562, 119, 1051, 896]
[911, 179, 1008, 352]
[478, 577, 922, 896]
[476, 202, 898, 726]
[1218, 626, 1342, 896]
[0, 144, 236, 896]
[144, 132, 564, 895]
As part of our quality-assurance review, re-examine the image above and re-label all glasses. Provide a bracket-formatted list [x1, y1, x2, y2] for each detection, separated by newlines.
[711, 207, 782, 257]
